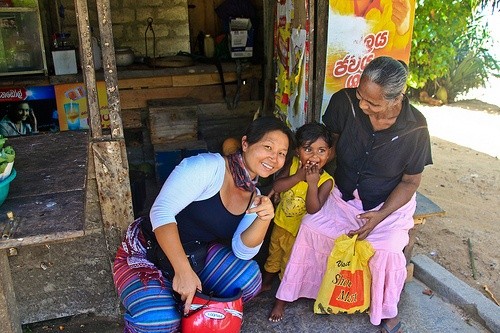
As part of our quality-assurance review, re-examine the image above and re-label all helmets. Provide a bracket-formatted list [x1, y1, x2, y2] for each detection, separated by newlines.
[180, 287, 243, 333]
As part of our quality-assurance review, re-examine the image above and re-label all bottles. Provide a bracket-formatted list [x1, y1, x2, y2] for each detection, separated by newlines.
[115, 46, 135, 68]
[52, 32, 75, 51]
[204, 34, 215, 59]
[90, 27, 102, 72]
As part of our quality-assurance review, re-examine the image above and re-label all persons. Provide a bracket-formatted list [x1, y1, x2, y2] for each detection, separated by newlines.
[262, 122, 333, 323]
[112, 116, 296, 333]
[0, 100, 37, 136]
[294, 55, 434, 333]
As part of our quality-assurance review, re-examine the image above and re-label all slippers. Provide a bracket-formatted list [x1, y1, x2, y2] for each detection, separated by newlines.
[383, 319, 401, 333]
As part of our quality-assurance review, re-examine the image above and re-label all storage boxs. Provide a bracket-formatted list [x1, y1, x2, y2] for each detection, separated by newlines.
[229, 29, 248, 48]
[54, 81, 112, 131]
[0, 16, 17, 29]
[227, 38, 253, 58]
[15, 50, 31, 67]
[51, 45, 78, 75]
[228, 16, 254, 31]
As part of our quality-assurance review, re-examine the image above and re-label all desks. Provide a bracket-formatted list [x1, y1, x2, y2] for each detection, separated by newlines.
[55, 56, 261, 131]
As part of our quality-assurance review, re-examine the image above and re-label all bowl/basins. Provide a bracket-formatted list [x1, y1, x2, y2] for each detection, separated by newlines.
[0, 168, 17, 206]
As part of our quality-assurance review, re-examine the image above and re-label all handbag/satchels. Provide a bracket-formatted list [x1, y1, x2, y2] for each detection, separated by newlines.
[141, 219, 209, 279]
[314, 232, 377, 314]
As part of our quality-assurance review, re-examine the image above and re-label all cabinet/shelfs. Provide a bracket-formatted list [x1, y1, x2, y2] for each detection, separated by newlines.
[0, 0, 55, 77]
